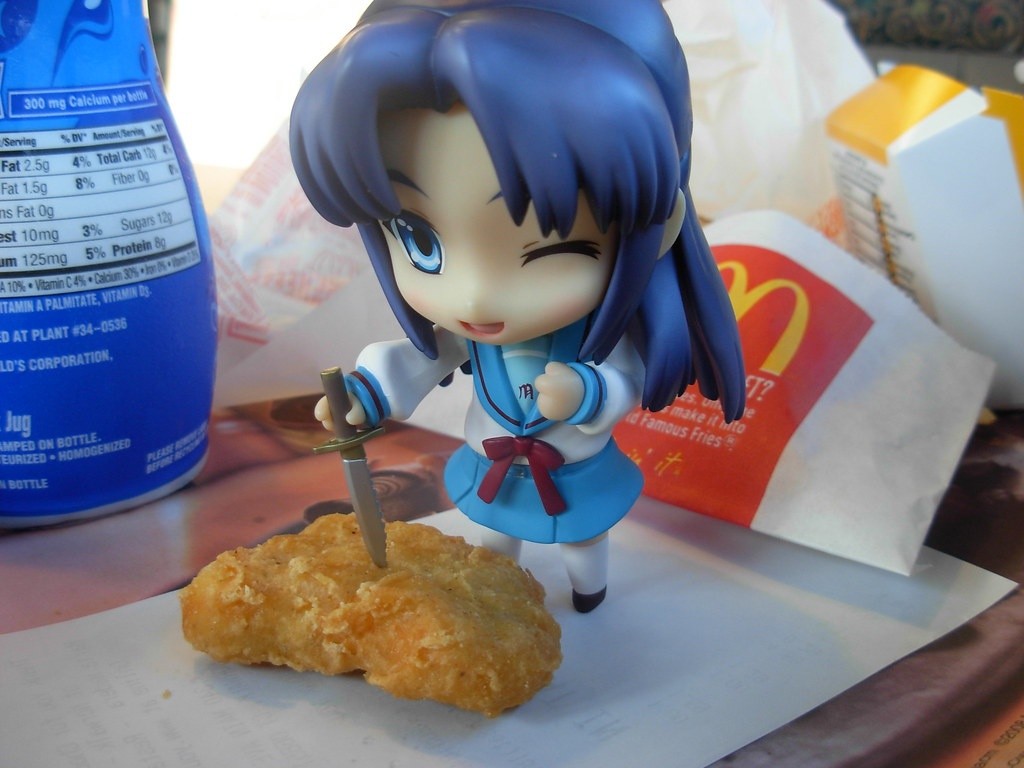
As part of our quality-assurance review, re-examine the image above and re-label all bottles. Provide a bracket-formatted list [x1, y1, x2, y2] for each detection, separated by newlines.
[0, 0, 220, 531]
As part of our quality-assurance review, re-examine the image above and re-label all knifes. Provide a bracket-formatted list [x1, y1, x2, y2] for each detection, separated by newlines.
[313, 366, 388, 569]
